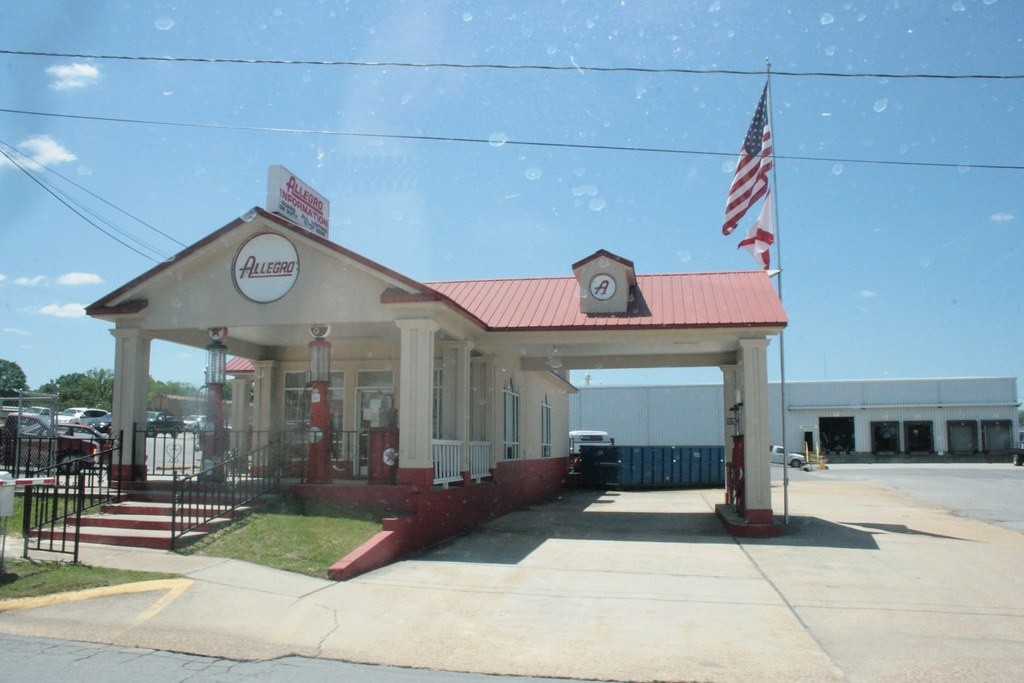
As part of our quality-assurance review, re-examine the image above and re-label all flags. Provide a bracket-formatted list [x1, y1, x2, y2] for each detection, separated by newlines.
[722, 81, 773, 236]
[736, 186, 775, 271]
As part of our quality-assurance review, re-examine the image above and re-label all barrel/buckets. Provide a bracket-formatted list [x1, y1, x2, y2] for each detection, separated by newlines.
[368, 427, 399, 485]
[0, 471, 15, 516]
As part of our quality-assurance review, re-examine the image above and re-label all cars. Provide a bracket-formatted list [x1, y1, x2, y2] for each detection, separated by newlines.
[26, 424, 111, 467]
[88, 414, 112, 433]
[22, 406, 52, 425]
[182, 415, 214, 432]
[47, 407, 112, 431]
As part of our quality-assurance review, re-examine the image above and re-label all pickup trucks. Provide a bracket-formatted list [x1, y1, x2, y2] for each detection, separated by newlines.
[770, 445, 807, 467]
[146, 411, 185, 438]
[0, 412, 99, 477]
